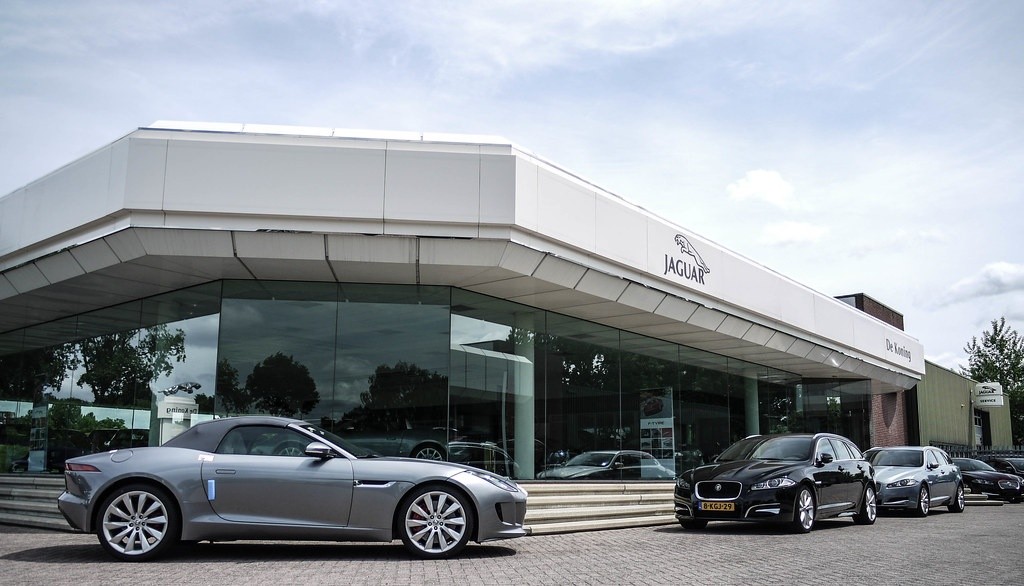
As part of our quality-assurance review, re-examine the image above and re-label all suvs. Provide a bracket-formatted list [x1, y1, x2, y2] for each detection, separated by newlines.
[536, 450, 677, 479]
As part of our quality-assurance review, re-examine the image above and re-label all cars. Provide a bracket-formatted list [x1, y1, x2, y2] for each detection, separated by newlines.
[252, 418, 520, 479]
[862, 446, 965, 517]
[46, 428, 149, 453]
[951, 457, 1024, 503]
[674, 433, 879, 534]
[56, 416, 533, 562]
